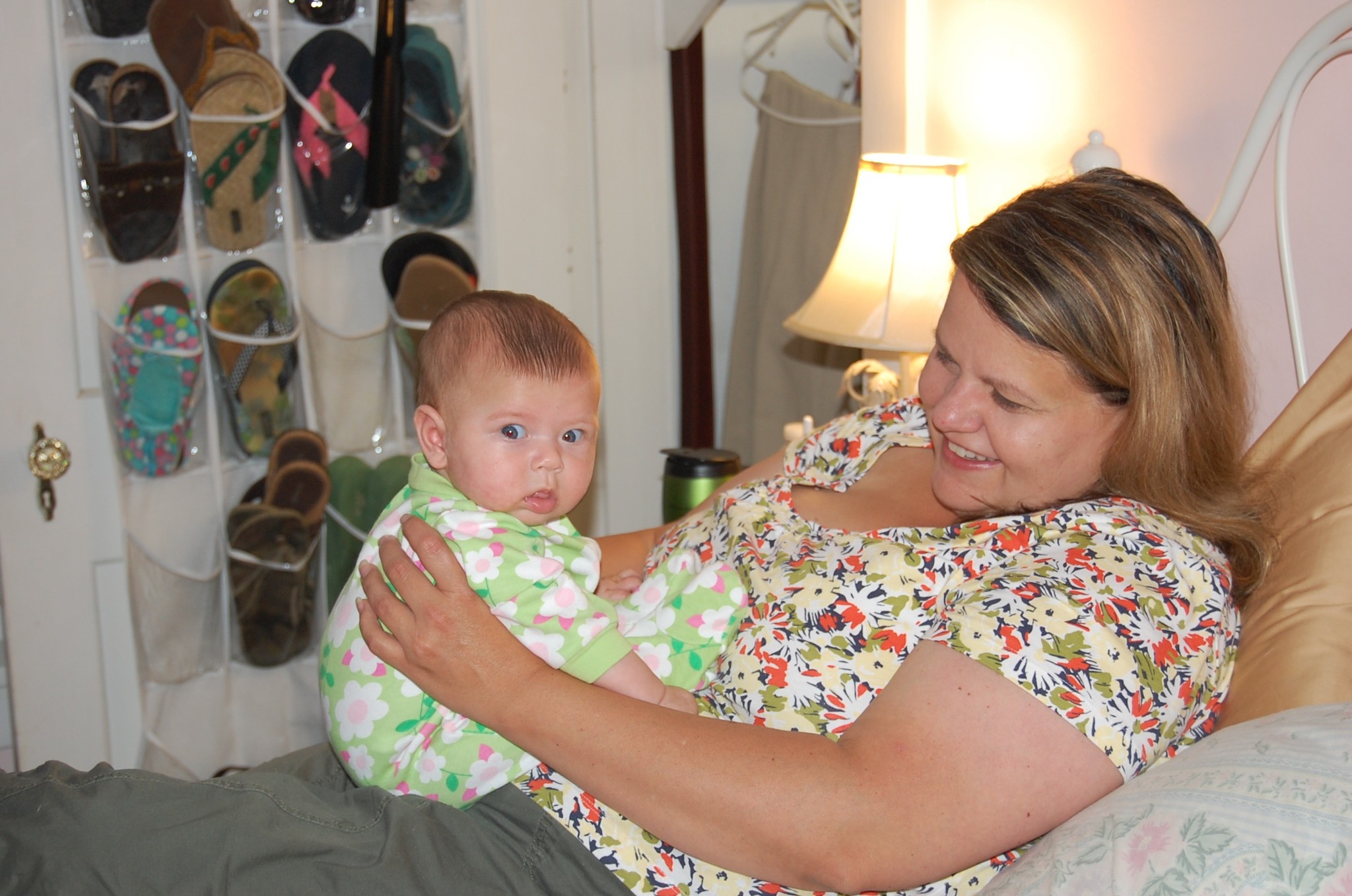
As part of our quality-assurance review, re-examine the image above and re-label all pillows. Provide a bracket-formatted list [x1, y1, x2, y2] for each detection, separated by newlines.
[983, 700, 1352, 896]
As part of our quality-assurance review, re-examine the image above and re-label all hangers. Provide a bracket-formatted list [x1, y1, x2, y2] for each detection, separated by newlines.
[736, 0, 864, 127]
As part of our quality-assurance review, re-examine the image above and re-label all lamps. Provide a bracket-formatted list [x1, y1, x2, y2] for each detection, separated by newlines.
[786, 148, 977, 404]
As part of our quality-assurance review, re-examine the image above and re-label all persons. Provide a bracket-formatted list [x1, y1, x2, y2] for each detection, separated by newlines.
[0, 167, 1269, 895]
[322, 289, 746, 808]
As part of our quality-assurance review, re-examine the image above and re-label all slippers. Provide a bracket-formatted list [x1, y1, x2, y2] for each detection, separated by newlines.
[205, 258, 299, 460]
[328, 455, 412, 614]
[380, 232, 477, 405]
[228, 504, 309, 667]
[113, 276, 203, 475]
[399, 21, 472, 226]
[239, 430, 329, 545]
[148, 0, 258, 105]
[283, 28, 377, 244]
[73, 59, 185, 266]
[183, 48, 283, 254]
